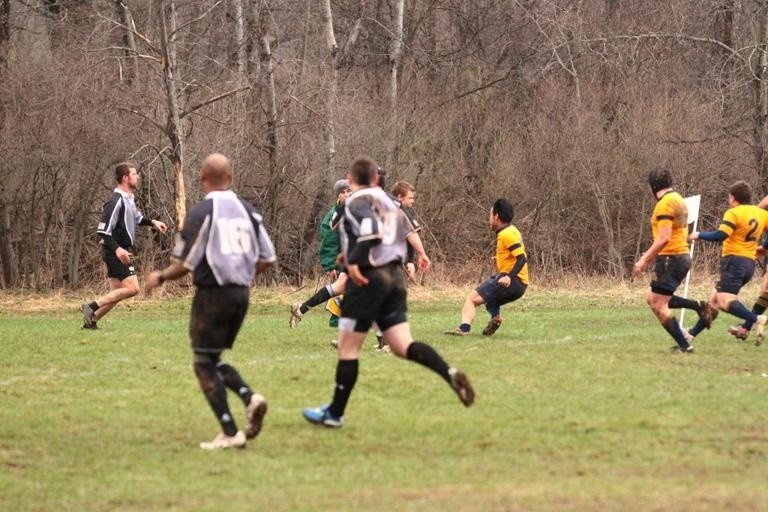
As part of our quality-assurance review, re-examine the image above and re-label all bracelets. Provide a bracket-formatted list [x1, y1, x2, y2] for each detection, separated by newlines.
[157, 267, 165, 285]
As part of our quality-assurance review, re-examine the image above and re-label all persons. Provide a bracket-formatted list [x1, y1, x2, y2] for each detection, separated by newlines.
[318, 176, 354, 329]
[328, 166, 422, 357]
[629, 168, 713, 356]
[726, 193, 767, 341]
[680, 180, 767, 347]
[442, 196, 530, 339]
[301, 153, 477, 429]
[287, 179, 431, 333]
[141, 152, 278, 453]
[81, 162, 167, 330]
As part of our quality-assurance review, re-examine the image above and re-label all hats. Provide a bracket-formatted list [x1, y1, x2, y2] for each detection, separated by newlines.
[648, 170, 673, 199]
[333, 179, 351, 198]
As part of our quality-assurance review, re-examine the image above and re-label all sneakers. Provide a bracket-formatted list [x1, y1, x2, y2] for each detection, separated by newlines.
[443, 327, 470, 336]
[483, 316, 501, 336]
[372, 343, 391, 355]
[290, 303, 305, 331]
[330, 339, 337, 347]
[244, 394, 268, 439]
[302, 404, 343, 429]
[670, 300, 768, 354]
[447, 368, 475, 407]
[82, 323, 98, 331]
[78, 303, 95, 325]
[199, 430, 248, 450]
